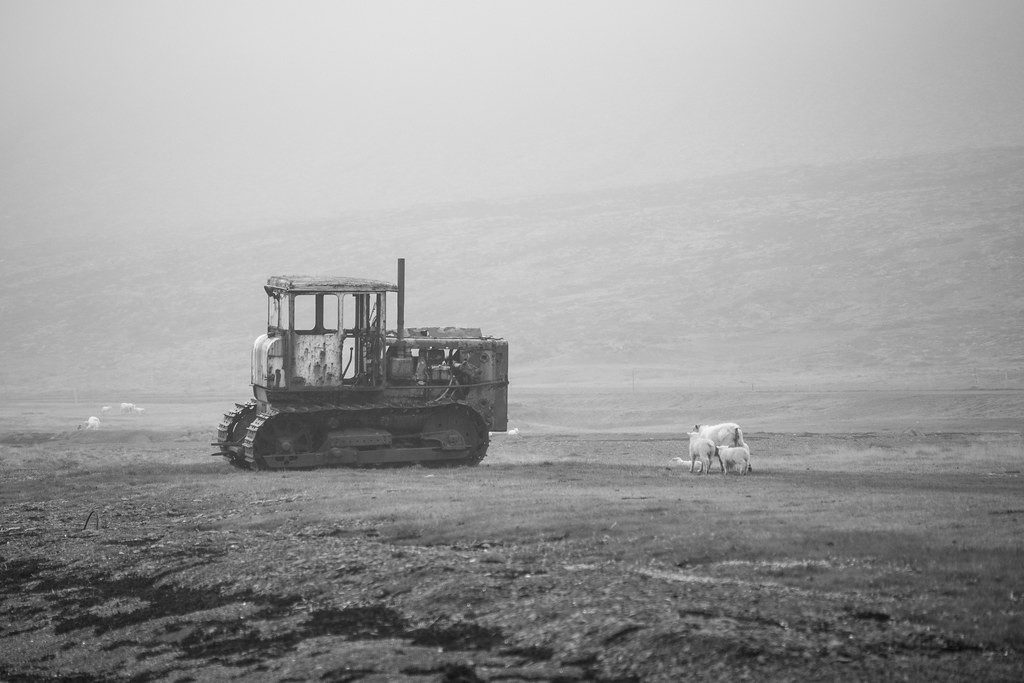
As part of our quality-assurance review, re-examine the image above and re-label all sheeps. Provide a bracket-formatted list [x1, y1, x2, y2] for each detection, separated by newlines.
[508, 427, 519, 435]
[668, 422, 750, 476]
[84, 402, 145, 431]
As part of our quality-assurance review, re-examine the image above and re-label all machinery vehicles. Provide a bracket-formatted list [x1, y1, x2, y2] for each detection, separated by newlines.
[211, 260, 510, 468]
